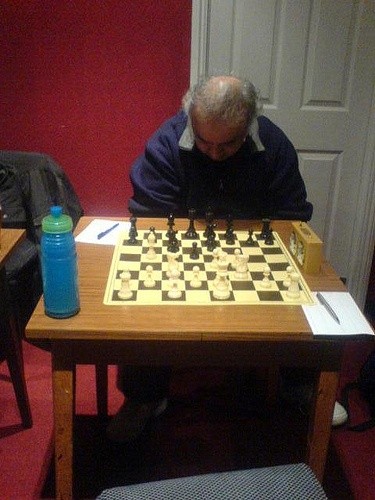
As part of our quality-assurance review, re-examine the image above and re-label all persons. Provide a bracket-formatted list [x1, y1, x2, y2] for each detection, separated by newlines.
[106, 75, 349, 443]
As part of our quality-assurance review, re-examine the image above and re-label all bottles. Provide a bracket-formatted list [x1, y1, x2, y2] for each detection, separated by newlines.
[41, 206, 81, 320]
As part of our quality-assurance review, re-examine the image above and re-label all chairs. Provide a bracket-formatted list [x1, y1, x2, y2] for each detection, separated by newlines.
[0, 151, 84, 330]
[94, 463, 329, 500]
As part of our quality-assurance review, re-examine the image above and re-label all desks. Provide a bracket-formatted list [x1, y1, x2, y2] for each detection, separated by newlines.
[26, 216, 375, 500]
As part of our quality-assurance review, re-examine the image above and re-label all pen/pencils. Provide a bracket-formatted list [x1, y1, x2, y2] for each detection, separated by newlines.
[97, 222, 119, 239]
[316, 292, 342, 326]
